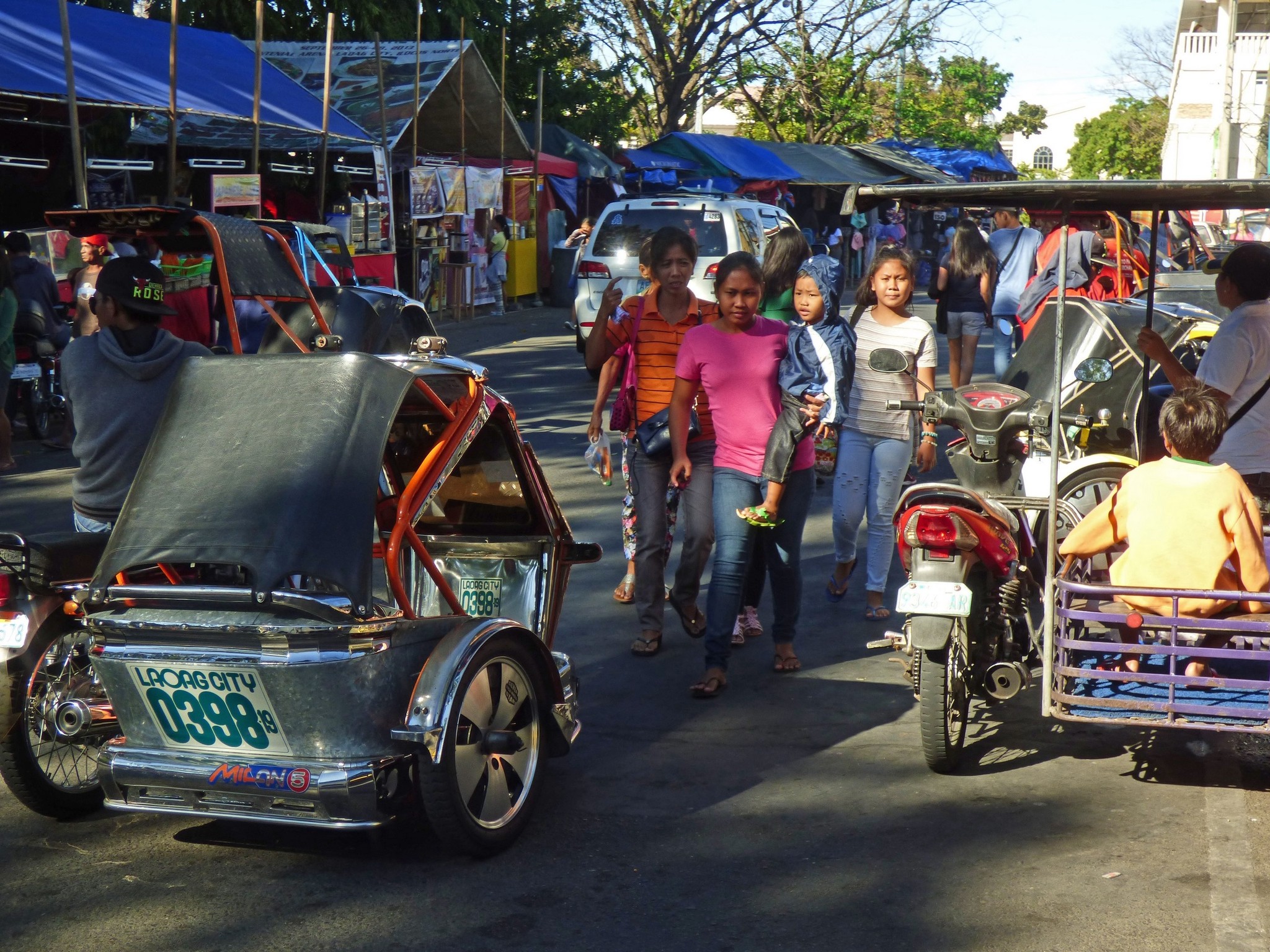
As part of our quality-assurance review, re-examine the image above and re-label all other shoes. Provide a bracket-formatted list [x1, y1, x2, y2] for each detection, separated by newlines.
[564, 321, 575, 330]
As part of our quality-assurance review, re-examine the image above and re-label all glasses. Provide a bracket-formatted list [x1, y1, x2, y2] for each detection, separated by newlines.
[89, 297, 110, 315]
[1091, 261, 1103, 274]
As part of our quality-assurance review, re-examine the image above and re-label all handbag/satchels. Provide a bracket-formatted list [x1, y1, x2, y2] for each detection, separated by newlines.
[610, 295, 647, 432]
[636, 404, 702, 455]
[810, 425, 838, 475]
[487, 257, 493, 265]
[584, 430, 614, 487]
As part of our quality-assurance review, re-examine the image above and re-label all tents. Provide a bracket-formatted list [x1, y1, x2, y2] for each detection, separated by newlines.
[0, 0, 1025, 234]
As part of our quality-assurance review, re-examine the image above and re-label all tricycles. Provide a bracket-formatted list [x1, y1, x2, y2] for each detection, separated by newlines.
[70, 354, 602, 860]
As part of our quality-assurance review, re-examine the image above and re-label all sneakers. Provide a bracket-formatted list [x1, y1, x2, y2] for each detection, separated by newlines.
[486, 308, 506, 315]
[902, 472, 917, 485]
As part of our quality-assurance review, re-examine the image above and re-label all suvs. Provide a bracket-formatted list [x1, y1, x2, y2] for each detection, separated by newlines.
[573, 190, 830, 384]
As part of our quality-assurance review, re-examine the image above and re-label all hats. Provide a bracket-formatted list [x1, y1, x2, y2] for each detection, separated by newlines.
[1222, 241, 1270, 295]
[1091, 234, 1119, 268]
[80, 233, 115, 256]
[984, 206, 1020, 218]
[96, 256, 180, 316]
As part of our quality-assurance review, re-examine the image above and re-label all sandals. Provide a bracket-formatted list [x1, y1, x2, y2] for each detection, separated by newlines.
[732, 605, 764, 644]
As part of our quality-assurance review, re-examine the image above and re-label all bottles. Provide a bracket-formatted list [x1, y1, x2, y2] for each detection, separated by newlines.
[520, 226, 527, 239]
[38, 253, 49, 267]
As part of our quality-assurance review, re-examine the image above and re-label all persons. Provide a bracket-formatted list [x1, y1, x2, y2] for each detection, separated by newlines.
[990, 206, 1044, 381]
[41, 235, 113, 453]
[1017, 230, 1118, 343]
[51, 209, 317, 332]
[1135, 224, 1175, 268]
[683, 217, 707, 251]
[485, 214, 511, 316]
[564, 217, 596, 331]
[825, 245, 937, 621]
[586, 227, 721, 657]
[667, 252, 815, 699]
[0, 231, 19, 471]
[930, 216, 1023, 266]
[1229, 219, 1254, 241]
[1260, 212, 1270, 242]
[757, 227, 811, 321]
[1136, 242, 1270, 497]
[734, 253, 857, 526]
[823, 218, 844, 260]
[928, 219, 998, 390]
[588, 234, 681, 604]
[1015, 226, 1089, 329]
[1058, 387, 1270, 688]
[5, 231, 74, 350]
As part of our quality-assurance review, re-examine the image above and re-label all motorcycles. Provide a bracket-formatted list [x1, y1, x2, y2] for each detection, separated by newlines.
[13, 299, 65, 437]
[867, 349, 1111, 773]
[2, 530, 126, 822]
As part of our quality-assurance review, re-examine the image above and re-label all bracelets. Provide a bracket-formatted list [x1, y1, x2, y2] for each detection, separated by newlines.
[921, 431, 938, 438]
[920, 439, 938, 446]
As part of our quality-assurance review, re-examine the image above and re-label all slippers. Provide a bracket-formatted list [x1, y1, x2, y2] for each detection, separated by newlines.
[669, 588, 707, 639]
[42, 438, 73, 449]
[691, 655, 800, 698]
[0, 455, 18, 471]
[1097, 657, 1130, 682]
[631, 632, 663, 656]
[736, 506, 786, 527]
[1184, 668, 1229, 688]
[826, 557, 889, 620]
[613, 574, 671, 602]
